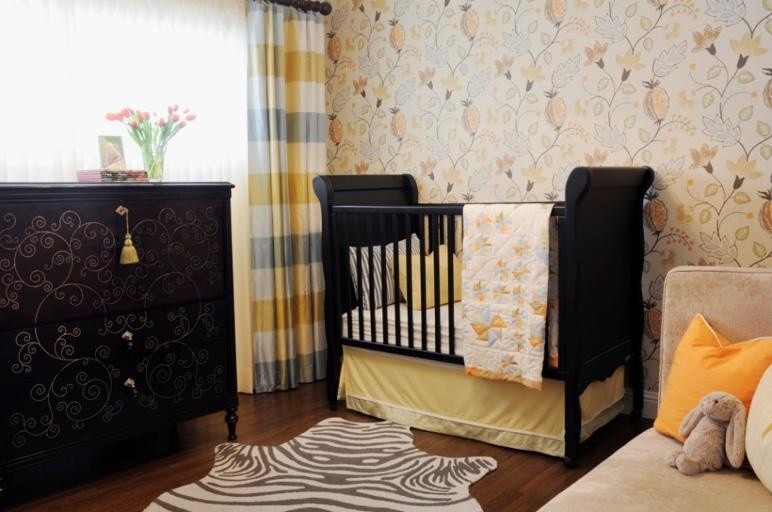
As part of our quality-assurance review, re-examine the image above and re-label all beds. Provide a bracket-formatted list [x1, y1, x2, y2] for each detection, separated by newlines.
[310, 165, 655, 471]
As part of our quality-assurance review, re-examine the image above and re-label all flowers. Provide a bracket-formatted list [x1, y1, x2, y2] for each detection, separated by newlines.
[106, 104, 196, 179]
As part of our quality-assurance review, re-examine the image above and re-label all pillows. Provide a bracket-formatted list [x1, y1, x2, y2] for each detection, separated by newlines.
[744, 365, 772, 493]
[388, 245, 462, 311]
[348, 232, 420, 309]
[653, 312, 771, 445]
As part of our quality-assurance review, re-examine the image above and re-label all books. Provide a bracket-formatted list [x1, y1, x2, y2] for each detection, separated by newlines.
[77, 170, 148, 182]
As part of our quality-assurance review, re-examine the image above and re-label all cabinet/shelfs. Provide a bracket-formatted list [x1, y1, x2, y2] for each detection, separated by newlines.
[0, 181, 239, 512]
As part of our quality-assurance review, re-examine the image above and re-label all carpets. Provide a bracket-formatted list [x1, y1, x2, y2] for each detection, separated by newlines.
[142, 415, 499, 512]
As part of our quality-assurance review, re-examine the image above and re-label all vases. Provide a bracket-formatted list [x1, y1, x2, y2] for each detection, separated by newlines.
[138, 140, 167, 183]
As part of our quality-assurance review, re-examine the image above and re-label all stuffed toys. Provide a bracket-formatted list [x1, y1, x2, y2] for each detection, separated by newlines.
[664, 390, 747, 475]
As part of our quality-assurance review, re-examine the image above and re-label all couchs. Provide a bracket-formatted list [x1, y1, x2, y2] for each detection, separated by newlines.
[536, 266, 772, 512]
